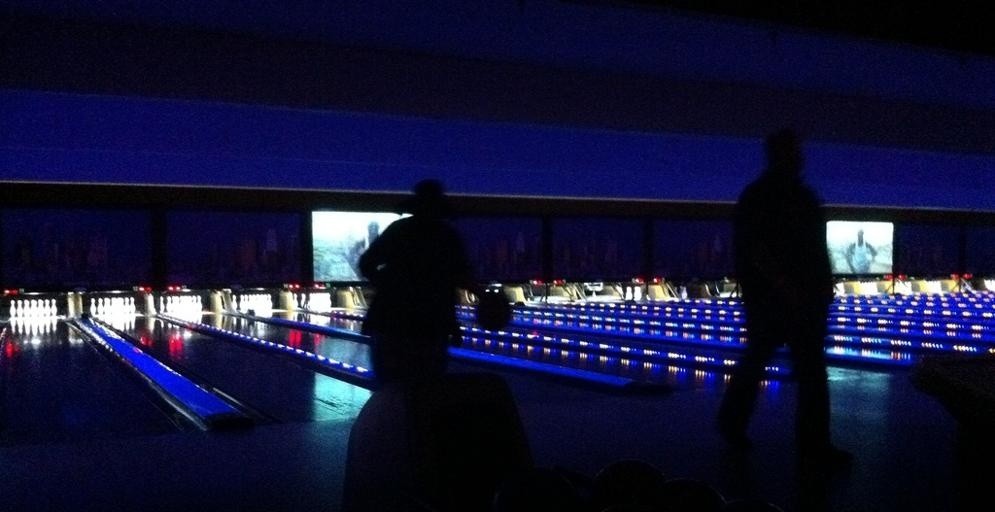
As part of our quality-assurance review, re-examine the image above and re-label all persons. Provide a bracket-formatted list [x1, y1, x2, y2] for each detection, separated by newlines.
[715, 128, 858, 468]
[356, 177, 492, 388]
